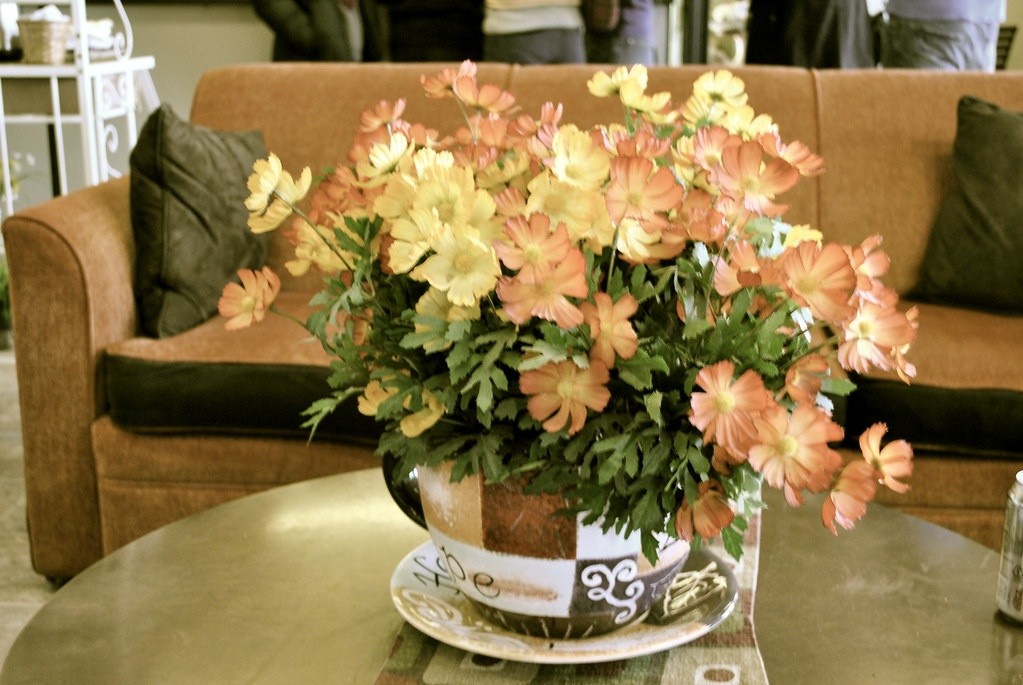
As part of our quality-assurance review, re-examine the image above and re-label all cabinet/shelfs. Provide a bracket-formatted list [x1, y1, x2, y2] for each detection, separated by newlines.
[0, 0, 154, 216]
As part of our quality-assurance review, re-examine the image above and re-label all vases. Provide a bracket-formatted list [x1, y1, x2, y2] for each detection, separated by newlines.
[376, 444, 692, 642]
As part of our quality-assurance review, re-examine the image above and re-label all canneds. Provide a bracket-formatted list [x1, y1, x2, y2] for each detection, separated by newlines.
[994, 469, 1023, 625]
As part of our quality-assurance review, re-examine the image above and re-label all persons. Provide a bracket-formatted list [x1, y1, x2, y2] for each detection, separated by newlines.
[251, 0, 1007, 71]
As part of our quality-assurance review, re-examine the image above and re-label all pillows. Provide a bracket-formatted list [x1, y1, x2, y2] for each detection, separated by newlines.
[900, 93, 1023, 318]
[128, 100, 269, 339]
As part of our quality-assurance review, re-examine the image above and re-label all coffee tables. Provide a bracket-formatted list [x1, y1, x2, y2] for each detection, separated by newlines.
[1, 466, 1022, 685]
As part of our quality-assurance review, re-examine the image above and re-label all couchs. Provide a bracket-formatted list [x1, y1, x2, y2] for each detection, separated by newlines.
[0, 61, 1023, 588]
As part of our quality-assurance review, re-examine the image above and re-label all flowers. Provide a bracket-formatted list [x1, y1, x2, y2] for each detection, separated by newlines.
[215, 62, 918, 573]
